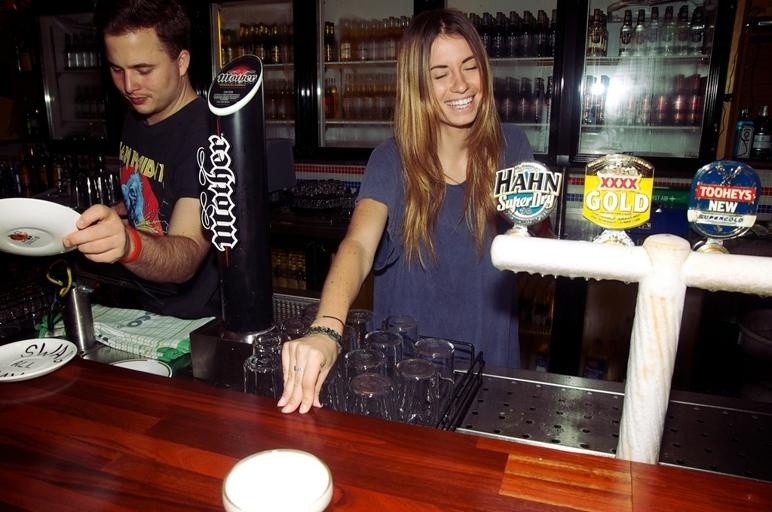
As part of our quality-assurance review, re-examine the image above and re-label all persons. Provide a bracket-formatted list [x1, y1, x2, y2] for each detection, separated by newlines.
[279, 4, 557, 419]
[60, 3, 213, 321]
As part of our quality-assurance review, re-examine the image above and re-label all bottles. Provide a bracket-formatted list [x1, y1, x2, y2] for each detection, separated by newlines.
[0, 29, 118, 197]
[733, 108, 754, 165]
[750, 106, 771, 168]
[210, 7, 711, 127]
[277, 179, 360, 223]
[564, 172, 772, 220]
[57, 285, 96, 350]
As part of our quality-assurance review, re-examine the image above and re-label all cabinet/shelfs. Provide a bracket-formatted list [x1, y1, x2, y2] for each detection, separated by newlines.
[43, 1, 739, 189]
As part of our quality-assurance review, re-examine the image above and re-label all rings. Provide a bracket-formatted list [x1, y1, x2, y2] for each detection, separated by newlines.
[292, 365, 303, 374]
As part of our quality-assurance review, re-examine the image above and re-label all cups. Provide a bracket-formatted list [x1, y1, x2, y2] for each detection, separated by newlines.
[246, 304, 456, 425]
[222, 447, 336, 511]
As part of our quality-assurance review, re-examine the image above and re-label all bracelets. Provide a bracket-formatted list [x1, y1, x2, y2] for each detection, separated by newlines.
[119, 224, 144, 263]
[302, 326, 344, 354]
[315, 315, 344, 327]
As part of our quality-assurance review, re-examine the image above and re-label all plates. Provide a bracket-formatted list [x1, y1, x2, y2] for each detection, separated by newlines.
[111, 358, 174, 379]
[0, 337, 78, 382]
[1, 195, 86, 258]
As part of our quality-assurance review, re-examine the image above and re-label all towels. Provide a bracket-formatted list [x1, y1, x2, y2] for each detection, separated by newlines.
[37, 301, 217, 365]
